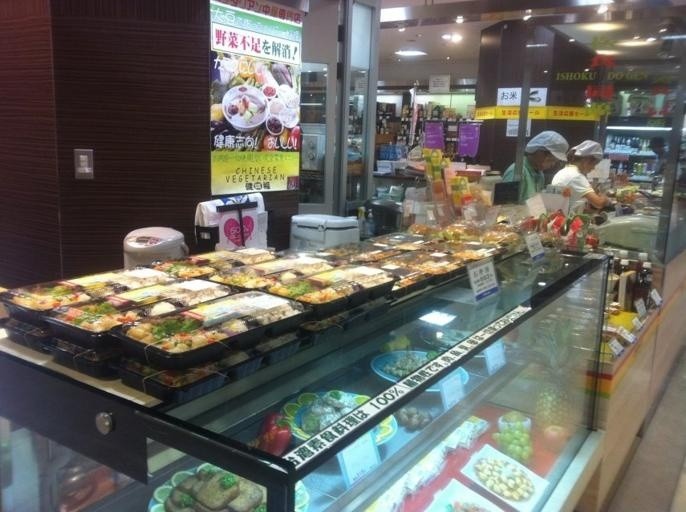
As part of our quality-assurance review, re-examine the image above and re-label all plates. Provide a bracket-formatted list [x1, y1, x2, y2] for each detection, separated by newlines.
[148, 464, 307, 510]
[369, 349, 471, 394]
[284, 389, 397, 447]
[423, 442, 550, 511]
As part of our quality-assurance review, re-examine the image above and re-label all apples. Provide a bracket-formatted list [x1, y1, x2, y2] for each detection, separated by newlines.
[544, 426, 571, 452]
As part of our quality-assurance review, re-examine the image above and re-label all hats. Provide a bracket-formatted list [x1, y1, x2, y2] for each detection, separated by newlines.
[524, 130, 568, 162]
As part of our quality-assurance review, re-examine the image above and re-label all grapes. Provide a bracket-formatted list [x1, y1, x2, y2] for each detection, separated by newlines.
[491, 421, 533, 466]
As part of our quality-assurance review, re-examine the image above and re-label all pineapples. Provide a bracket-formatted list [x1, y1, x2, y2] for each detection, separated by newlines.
[529, 319, 579, 430]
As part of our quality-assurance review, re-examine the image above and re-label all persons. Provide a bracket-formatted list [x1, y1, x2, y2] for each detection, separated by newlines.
[551, 139, 610, 211]
[503, 131, 569, 204]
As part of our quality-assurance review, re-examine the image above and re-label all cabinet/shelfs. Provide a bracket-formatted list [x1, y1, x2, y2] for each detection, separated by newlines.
[0, 116, 686, 509]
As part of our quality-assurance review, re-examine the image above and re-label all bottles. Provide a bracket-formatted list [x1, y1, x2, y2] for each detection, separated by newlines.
[614, 135, 649, 151]
[607, 249, 653, 308]
[617, 160, 627, 176]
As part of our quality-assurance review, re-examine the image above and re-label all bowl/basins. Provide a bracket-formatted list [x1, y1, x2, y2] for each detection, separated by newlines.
[498, 410, 532, 434]
[222, 81, 298, 138]
